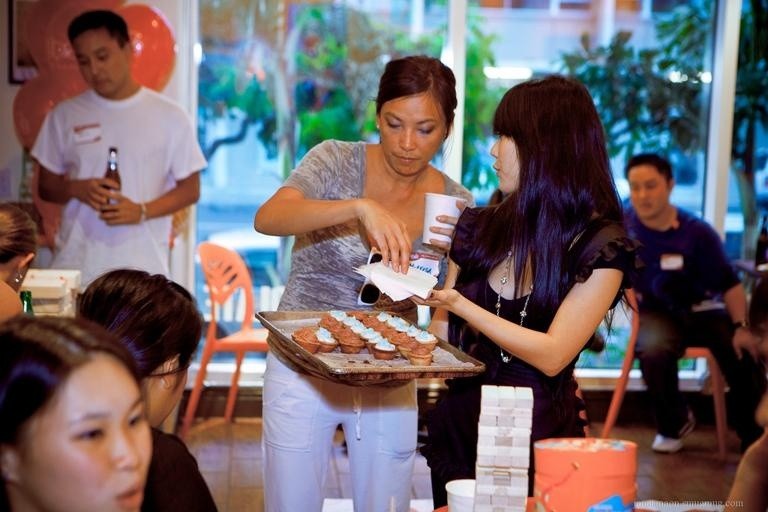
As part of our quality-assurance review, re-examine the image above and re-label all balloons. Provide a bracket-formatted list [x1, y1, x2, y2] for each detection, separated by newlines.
[14, 3, 176, 249]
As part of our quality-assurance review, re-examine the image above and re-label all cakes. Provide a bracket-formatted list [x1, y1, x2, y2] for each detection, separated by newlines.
[290, 310, 439, 369]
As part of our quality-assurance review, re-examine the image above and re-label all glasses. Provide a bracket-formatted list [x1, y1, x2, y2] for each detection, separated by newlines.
[356, 247, 382, 306]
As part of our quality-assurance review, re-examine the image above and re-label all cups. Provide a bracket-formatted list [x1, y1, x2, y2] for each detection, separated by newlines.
[421, 193, 467, 246]
[445, 479, 476, 512]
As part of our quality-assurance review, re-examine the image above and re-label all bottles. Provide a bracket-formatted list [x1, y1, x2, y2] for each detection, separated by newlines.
[20, 290, 35, 314]
[100, 147, 122, 214]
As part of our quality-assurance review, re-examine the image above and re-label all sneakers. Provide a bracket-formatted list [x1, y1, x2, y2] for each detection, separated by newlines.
[651, 410, 696, 452]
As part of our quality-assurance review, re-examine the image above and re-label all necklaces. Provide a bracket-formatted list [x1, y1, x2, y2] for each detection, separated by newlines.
[494, 230, 584, 364]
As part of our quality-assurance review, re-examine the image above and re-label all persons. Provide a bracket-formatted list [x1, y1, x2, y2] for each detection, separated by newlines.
[254, 55, 477, 512]
[78, 270, 218, 512]
[0, 314, 153, 512]
[31, 8, 207, 294]
[0, 203, 40, 324]
[724, 276, 768, 512]
[622, 154, 763, 455]
[410, 75, 647, 509]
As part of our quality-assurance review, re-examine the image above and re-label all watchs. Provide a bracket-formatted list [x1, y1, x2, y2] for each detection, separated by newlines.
[733, 320, 750, 329]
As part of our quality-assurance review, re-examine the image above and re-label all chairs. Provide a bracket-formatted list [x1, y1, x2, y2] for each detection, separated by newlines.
[181, 243, 269, 443]
[594, 291, 730, 465]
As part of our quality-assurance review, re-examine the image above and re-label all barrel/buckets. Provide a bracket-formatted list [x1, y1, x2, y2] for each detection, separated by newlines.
[531, 436, 639, 512]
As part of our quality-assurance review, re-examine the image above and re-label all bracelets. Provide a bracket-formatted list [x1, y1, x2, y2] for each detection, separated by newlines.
[139, 202, 146, 224]
